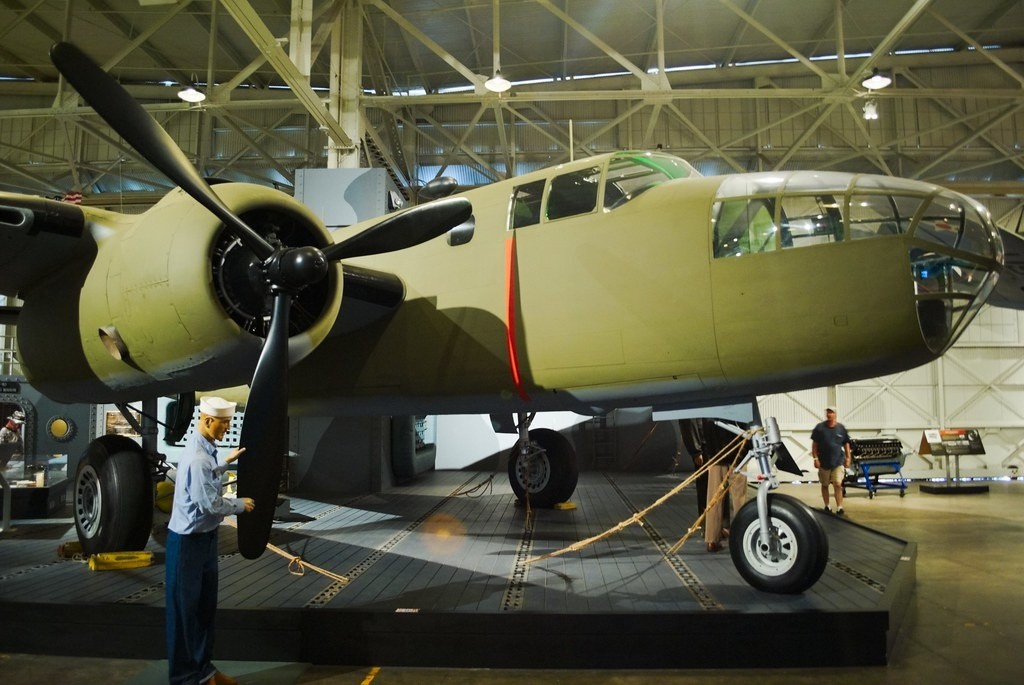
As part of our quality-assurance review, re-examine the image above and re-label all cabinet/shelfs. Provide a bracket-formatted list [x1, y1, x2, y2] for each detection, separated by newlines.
[0, 452, 69, 521]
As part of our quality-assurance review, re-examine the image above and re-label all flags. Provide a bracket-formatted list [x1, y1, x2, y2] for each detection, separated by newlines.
[45, 191, 82, 207]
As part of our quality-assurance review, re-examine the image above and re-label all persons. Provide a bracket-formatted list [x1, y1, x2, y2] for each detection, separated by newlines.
[811, 405, 851, 516]
[0, 410, 26, 453]
[166, 394, 255, 684]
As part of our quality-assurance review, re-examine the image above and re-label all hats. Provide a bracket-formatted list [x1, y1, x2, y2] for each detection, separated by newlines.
[825, 406, 836, 413]
[199, 395, 237, 417]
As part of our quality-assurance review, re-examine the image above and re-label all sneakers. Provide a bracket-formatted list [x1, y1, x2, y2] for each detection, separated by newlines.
[824, 504, 832, 511]
[836, 506, 844, 514]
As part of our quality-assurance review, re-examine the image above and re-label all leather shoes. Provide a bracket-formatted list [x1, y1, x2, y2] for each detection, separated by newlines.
[200, 670, 237, 684]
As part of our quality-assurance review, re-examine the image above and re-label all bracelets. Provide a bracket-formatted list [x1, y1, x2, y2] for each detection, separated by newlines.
[813, 457, 819, 460]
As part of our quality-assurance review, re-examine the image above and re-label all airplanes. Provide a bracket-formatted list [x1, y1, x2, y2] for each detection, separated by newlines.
[2, 18, 1010, 604]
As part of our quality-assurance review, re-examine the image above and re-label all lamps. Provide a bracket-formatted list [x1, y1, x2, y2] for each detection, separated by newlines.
[177, 73, 206, 107]
[861, 64, 893, 94]
[482, 69, 513, 103]
